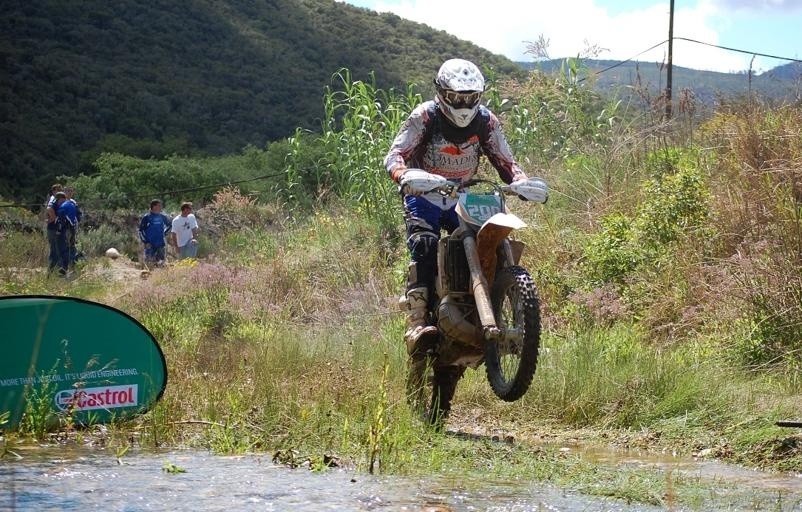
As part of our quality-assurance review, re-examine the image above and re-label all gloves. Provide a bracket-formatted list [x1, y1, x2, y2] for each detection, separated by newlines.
[398, 168, 425, 198]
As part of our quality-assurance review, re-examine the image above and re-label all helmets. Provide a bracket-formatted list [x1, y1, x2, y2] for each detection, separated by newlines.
[433, 58, 485, 128]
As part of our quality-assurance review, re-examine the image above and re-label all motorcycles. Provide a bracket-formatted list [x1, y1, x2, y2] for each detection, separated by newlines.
[396, 167, 549, 433]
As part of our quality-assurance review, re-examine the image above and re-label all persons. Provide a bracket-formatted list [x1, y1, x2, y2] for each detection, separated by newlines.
[383, 56, 548, 354]
[172, 202, 200, 258]
[46, 183, 86, 275]
[139, 199, 172, 262]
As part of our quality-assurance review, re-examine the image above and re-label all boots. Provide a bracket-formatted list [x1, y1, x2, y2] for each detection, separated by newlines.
[398, 287, 438, 359]
